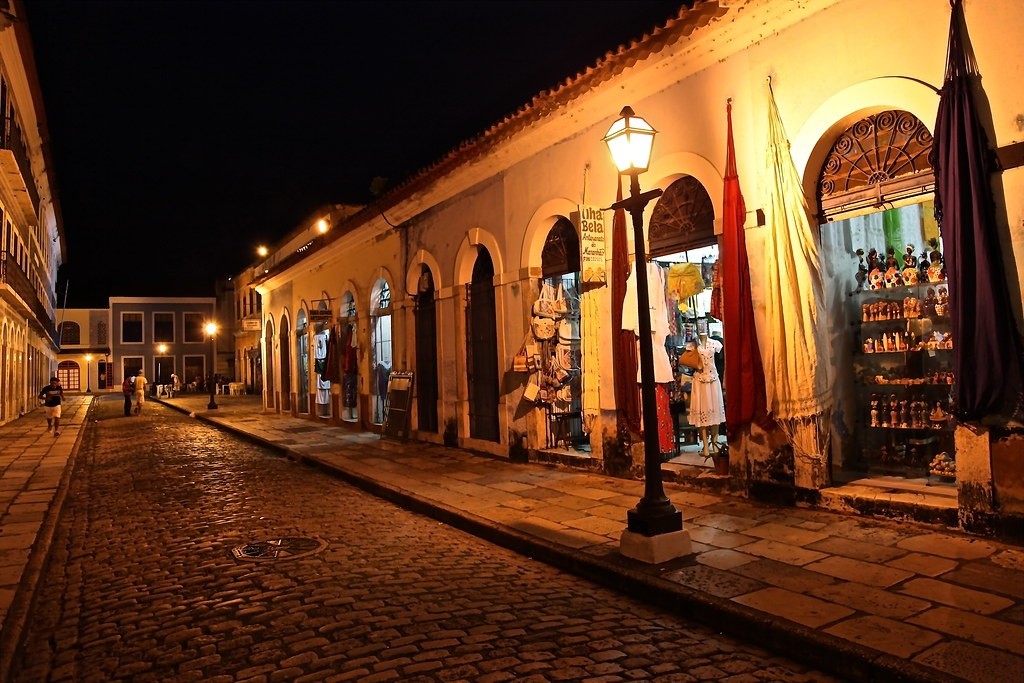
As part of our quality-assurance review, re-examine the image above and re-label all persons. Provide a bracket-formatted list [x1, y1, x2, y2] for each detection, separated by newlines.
[854, 237, 955, 468]
[686, 332, 728, 459]
[122, 370, 229, 417]
[38, 377, 66, 437]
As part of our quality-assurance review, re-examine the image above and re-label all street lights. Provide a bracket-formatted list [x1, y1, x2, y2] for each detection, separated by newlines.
[158, 345, 166, 387]
[86, 355, 92, 393]
[602, 103, 686, 535]
[205, 323, 219, 409]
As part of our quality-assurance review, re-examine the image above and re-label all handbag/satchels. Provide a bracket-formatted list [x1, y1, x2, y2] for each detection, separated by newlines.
[678, 340, 704, 370]
[514, 282, 581, 411]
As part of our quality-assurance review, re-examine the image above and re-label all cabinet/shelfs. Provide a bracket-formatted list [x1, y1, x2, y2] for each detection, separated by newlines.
[850, 281, 954, 477]
